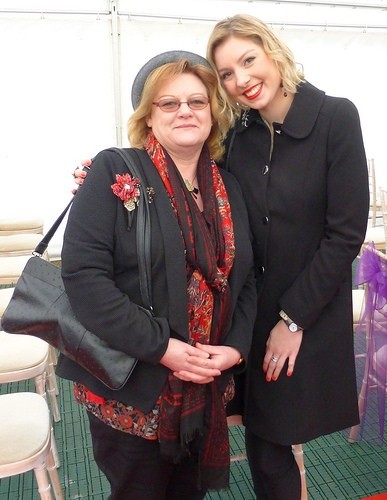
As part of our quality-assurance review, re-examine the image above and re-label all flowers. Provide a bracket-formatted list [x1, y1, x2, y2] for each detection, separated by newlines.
[112, 173, 140, 210]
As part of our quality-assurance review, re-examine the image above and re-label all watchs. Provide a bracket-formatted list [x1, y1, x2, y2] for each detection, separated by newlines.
[235, 357, 245, 366]
[279, 310, 303, 332]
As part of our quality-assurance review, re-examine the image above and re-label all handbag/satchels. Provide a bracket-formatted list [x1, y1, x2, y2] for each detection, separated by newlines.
[0, 255, 154, 392]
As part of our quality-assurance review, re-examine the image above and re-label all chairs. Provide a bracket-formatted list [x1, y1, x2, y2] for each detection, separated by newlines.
[0, 213, 63, 425]
[353, 289, 387, 359]
[365, 225, 386, 250]
[347, 244, 387, 443]
[0, 391, 65, 500]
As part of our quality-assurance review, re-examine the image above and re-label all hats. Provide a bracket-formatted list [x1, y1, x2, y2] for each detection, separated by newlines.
[131, 50, 213, 112]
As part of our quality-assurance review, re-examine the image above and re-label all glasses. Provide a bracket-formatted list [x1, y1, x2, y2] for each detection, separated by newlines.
[152, 96, 210, 112]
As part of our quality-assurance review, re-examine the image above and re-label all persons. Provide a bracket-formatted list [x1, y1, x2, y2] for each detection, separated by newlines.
[54, 50, 256, 500]
[71, 15, 369, 500]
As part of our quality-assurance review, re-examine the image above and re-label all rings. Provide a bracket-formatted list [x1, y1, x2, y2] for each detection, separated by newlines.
[271, 356, 278, 362]
